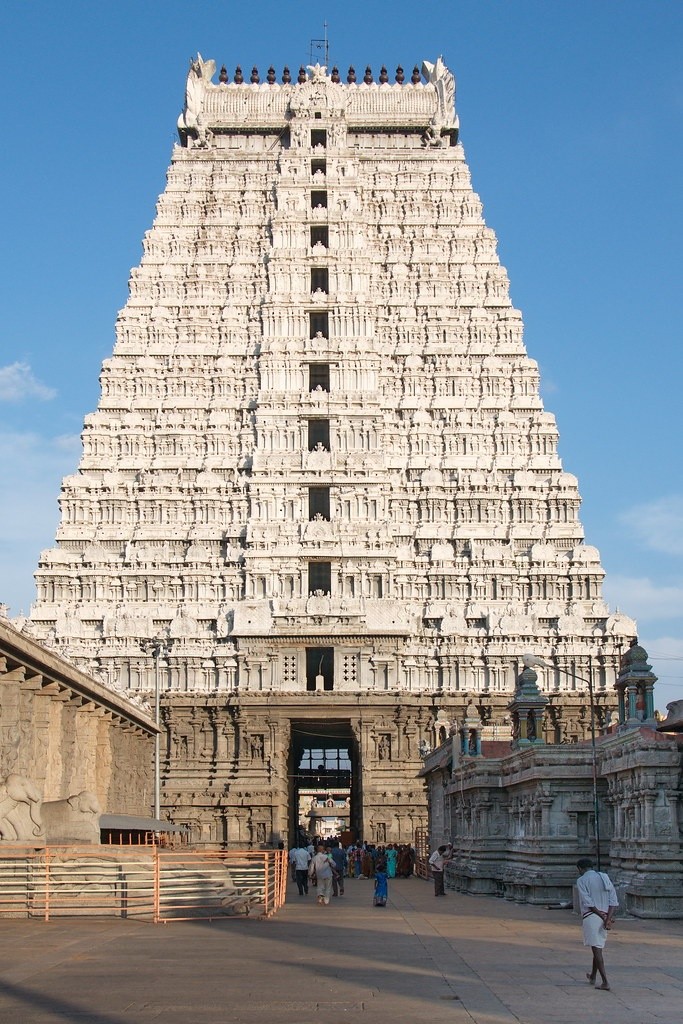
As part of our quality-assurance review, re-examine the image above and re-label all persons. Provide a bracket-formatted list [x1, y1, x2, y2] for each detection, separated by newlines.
[329, 840, 348, 896]
[322, 848, 340, 878]
[277, 843, 288, 869]
[576, 859, 619, 991]
[291, 844, 312, 894]
[372, 864, 387, 906]
[441, 842, 454, 864]
[307, 845, 337, 905]
[428, 845, 447, 895]
[286, 834, 413, 877]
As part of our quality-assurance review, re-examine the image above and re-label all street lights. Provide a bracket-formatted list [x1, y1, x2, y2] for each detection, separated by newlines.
[137, 636, 163, 841]
[521, 652, 601, 872]
